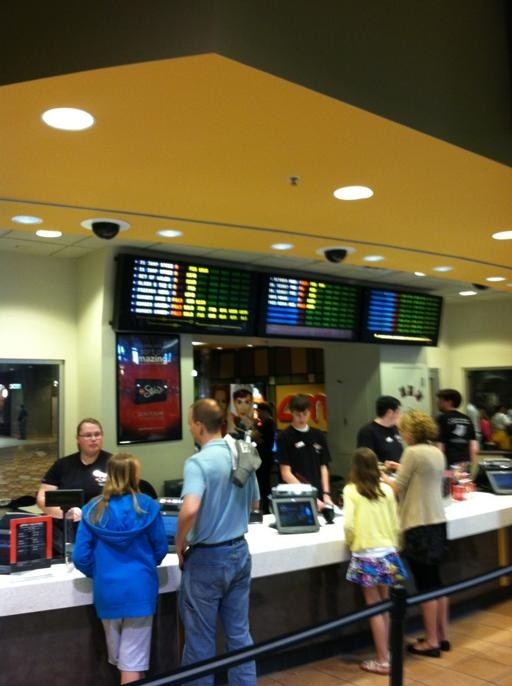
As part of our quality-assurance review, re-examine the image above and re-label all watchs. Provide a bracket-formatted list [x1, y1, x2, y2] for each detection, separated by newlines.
[322, 491, 332, 496]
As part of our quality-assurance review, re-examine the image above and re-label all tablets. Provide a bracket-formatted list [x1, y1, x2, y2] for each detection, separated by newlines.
[272, 497, 320, 534]
[486, 470, 512, 494]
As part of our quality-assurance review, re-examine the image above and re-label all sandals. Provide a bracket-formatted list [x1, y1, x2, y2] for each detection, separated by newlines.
[361, 659, 391, 675]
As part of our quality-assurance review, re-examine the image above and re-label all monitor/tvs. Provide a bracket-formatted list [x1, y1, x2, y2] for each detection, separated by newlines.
[160, 510, 180, 553]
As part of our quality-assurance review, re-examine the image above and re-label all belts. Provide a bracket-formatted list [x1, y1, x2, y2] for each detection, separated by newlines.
[190, 534, 245, 549]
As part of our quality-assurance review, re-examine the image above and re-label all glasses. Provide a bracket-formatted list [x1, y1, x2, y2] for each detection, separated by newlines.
[237, 399, 249, 405]
[216, 398, 227, 403]
[78, 432, 103, 440]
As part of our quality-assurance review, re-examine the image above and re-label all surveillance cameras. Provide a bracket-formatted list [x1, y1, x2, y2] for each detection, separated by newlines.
[472, 283, 490, 291]
[323, 249, 347, 264]
[91, 221, 119, 240]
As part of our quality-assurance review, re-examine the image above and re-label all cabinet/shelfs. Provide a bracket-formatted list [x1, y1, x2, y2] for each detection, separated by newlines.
[0, 489, 512, 686]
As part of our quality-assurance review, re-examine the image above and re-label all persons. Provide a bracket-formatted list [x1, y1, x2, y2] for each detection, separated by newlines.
[36, 419, 124, 544]
[252, 403, 277, 515]
[435, 388, 479, 481]
[212, 385, 240, 438]
[278, 394, 340, 516]
[343, 448, 412, 674]
[467, 403, 482, 442]
[177, 398, 258, 686]
[491, 405, 512, 450]
[477, 405, 492, 442]
[233, 384, 255, 430]
[73, 452, 168, 685]
[382, 413, 450, 657]
[356, 396, 405, 464]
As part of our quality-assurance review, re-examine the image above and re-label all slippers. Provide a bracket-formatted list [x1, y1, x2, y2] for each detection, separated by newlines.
[406, 642, 441, 657]
[417, 636, 450, 651]
